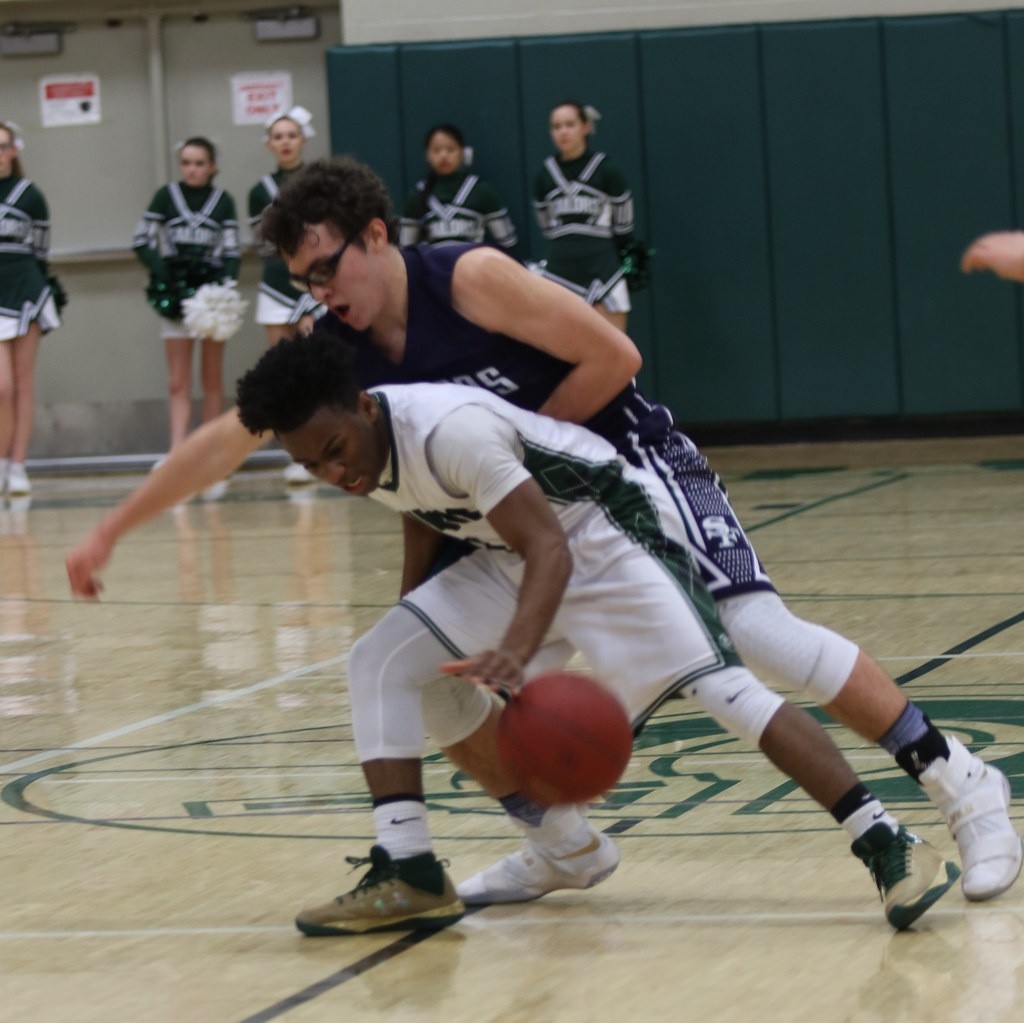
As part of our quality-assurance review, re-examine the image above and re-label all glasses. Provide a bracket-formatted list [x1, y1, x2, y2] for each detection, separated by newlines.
[284, 226, 366, 294]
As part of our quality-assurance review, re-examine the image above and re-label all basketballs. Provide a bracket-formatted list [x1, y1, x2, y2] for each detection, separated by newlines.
[500, 671, 635, 806]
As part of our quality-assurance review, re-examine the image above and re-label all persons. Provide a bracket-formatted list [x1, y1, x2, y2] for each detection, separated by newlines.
[63, 157, 1023, 909]
[233, 334, 962, 938]
[246, 105, 362, 489]
[531, 97, 638, 333]
[396, 121, 524, 263]
[127, 133, 242, 508]
[0, 120, 73, 504]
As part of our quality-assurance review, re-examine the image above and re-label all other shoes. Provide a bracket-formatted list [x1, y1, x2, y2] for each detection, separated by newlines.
[286, 461, 319, 483]
[0, 472, 31, 495]
[202, 479, 231, 500]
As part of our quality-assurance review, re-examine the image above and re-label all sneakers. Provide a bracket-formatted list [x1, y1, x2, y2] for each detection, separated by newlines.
[918, 734, 1022, 902]
[456, 830, 619, 903]
[296, 846, 466, 936]
[851, 822, 961, 930]
